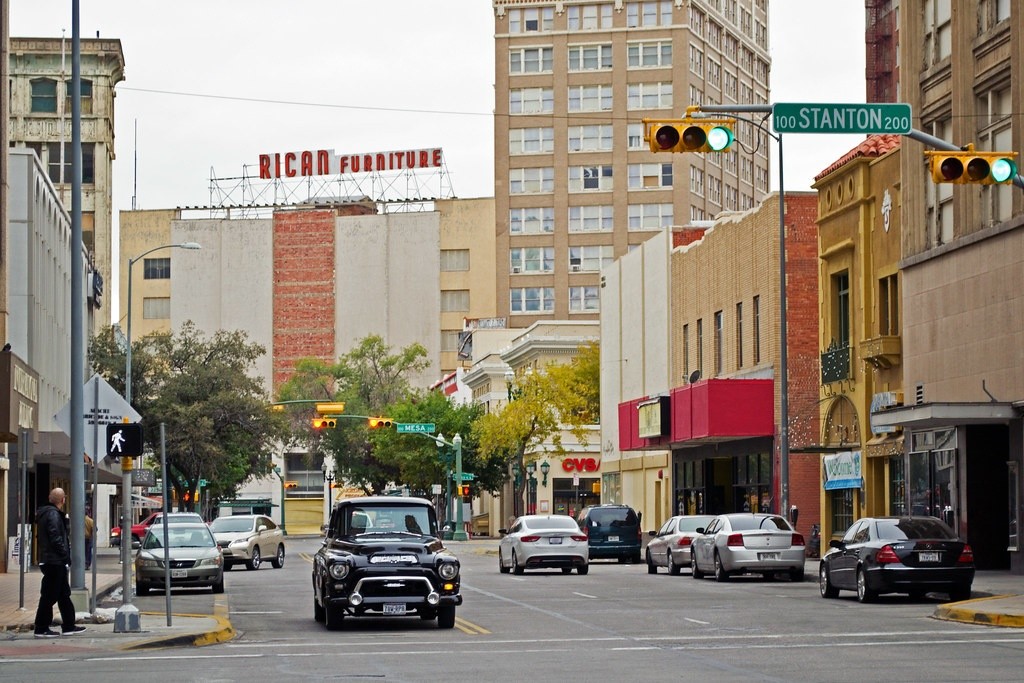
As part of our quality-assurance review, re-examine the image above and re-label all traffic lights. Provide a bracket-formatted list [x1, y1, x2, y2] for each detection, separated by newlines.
[285, 483, 298, 489]
[369, 419, 392, 429]
[462, 487, 470, 496]
[649, 123, 734, 153]
[105, 423, 144, 456]
[933, 155, 1018, 184]
[312, 419, 336, 429]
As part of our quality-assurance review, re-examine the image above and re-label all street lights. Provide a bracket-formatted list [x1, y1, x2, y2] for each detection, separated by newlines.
[112, 241, 203, 634]
[321, 462, 339, 519]
[436, 432, 463, 540]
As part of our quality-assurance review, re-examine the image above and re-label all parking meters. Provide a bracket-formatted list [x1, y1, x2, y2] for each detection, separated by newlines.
[790, 506, 798, 531]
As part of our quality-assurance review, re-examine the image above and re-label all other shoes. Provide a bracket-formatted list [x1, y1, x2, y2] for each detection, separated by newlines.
[60, 624, 86, 635]
[85, 565, 90, 570]
[34, 626, 60, 638]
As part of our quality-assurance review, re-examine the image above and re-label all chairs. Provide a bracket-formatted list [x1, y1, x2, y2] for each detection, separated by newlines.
[190, 532, 204, 544]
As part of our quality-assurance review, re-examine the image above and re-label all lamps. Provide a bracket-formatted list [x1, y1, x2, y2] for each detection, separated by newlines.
[540, 459, 552, 487]
[511, 462, 523, 489]
[525, 458, 537, 484]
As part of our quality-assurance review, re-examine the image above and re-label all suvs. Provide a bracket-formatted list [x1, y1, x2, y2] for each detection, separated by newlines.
[576, 503, 643, 565]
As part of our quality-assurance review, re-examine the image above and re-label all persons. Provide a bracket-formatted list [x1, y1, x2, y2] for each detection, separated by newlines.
[34, 488, 86, 637]
[84, 509, 94, 570]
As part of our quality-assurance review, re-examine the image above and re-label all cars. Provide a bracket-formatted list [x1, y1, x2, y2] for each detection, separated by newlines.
[110, 511, 166, 546]
[689, 511, 807, 582]
[311, 494, 464, 631]
[134, 512, 230, 596]
[209, 514, 285, 570]
[499, 514, 589, 576]
[645, 513, 722, 576]
[817, 515, 976, 604]
